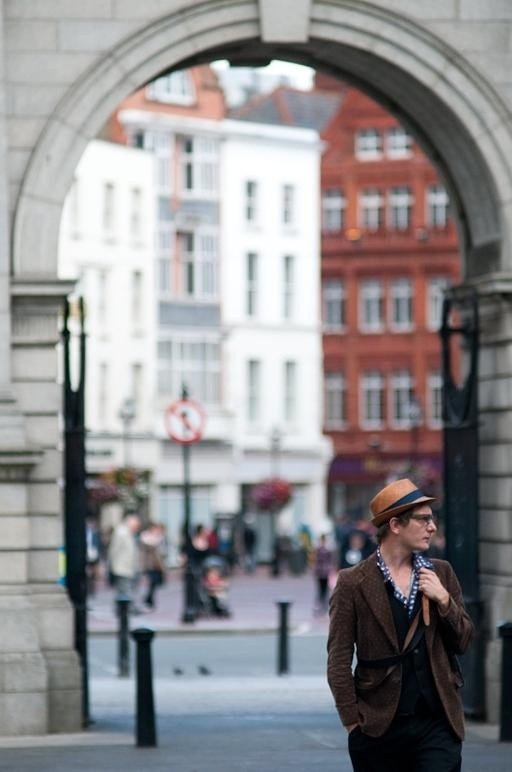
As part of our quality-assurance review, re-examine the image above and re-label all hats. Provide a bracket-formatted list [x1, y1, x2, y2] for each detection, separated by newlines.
[368, 478, 438, 528]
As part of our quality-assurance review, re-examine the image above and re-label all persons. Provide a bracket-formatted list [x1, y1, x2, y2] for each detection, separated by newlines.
[327, 478, 477, 771]
[55, 509, 257, 679]
[299, 506, 379, 615]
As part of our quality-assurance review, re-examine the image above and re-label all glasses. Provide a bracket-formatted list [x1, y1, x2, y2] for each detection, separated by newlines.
[401, 513, 436, 525]
